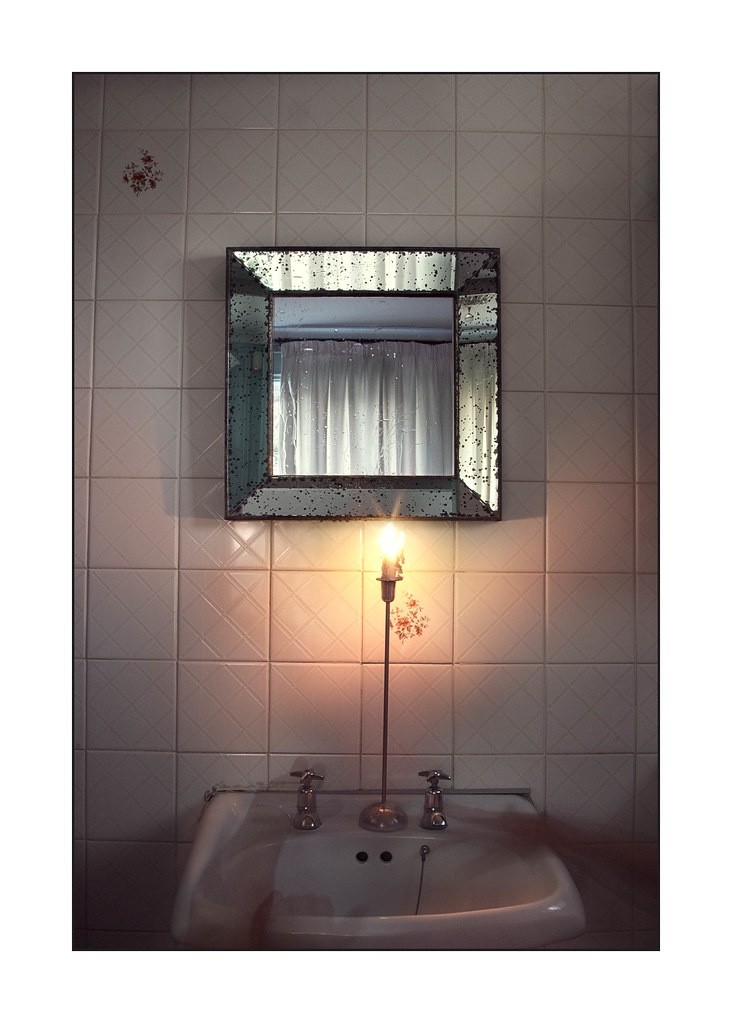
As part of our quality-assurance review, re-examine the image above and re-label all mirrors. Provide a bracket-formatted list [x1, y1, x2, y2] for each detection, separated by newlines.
[222, 243, 503, 524]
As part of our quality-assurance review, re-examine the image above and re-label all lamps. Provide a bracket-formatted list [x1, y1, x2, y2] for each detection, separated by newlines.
[361, 521, 408, 831]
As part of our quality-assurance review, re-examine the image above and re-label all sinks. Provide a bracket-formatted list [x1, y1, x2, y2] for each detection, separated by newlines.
[173, 793, 586, 949]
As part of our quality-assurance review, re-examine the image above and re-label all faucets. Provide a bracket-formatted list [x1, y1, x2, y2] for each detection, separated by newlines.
[418, 770, 452, 830]
[290, 769, 324, 830]
